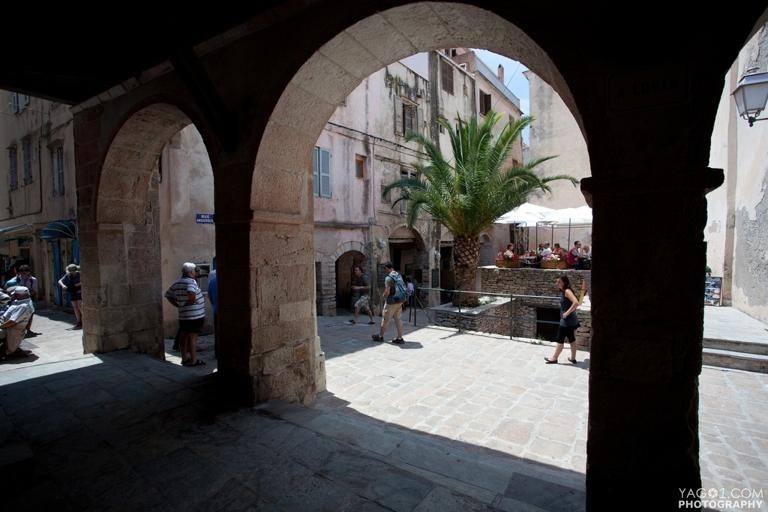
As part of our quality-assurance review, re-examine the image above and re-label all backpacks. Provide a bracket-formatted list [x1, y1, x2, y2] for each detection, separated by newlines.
[387, 272, 407, 304]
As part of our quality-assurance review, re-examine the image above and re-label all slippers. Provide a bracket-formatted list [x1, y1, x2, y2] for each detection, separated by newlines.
[181, 358, 191, 364]
[190, 359, 207, 366]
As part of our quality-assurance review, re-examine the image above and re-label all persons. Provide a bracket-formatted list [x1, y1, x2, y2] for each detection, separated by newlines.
[404, 278, 415, 296]
[497, 240, 593, 269]
[58, 263, 83, 330]
[348, 265, 376, 324]
[372, 261, 406, 344]
[544, 275, 583, 365]
[0, 254, 39, 362]
[164, 255, 218, 369]
[40, 238, 67, 306]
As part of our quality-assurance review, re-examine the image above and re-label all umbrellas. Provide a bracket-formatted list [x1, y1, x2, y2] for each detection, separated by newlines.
[494, 199, 594, 250]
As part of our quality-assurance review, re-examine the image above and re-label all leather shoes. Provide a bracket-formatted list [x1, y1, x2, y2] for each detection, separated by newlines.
[544, 357, 557, 363]
[568, 357, 576, 363]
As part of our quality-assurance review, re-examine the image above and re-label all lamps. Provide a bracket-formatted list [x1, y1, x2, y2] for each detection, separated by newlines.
[730, 66, 768, 127]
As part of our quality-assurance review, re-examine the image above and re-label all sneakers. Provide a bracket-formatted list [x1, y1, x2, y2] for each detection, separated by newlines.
[368, 321, 376, 325]
[349, 319, 355, 324]
[392, 338, 406, 345]
[73, 322, 83, 329]
[372, 334, 384, 342]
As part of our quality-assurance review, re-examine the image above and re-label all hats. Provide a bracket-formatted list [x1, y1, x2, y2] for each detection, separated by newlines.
[15, 287, 29, 295]
[67, 264, 81, 273]
